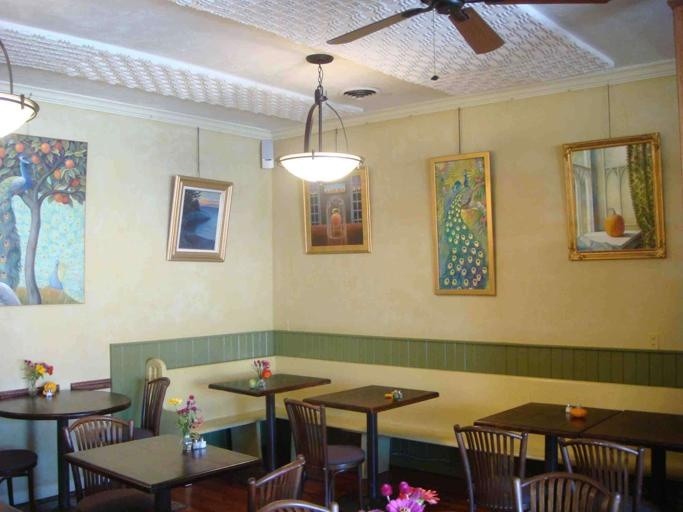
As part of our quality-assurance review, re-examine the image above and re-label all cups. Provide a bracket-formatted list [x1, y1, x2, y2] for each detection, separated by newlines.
[247, 378, 257, 390]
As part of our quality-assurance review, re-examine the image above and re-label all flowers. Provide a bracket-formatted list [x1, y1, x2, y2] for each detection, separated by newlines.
[22, 357, 54, 390]
[250, 358, 271, 382]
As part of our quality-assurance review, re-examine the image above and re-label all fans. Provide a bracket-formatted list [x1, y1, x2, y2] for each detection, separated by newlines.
[325, 1, 609, 56]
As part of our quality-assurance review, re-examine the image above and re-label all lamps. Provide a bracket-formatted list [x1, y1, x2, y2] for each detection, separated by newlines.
[277, 55, 364, 186]
[0, 41, 39, 140]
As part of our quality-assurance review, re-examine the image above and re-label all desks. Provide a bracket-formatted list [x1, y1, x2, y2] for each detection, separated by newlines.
[472, 402, 682, 512]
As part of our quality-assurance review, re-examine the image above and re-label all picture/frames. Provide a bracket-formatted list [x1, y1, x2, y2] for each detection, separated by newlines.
[428, 150, 497, 295]
[560, 132, 668, 262]
[165, 174, 234, 263]
[300, 166, 372, 254]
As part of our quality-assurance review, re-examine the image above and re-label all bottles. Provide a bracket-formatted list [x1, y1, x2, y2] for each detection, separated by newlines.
[563, 402, 587, 420]
[192, 437, 206, 451]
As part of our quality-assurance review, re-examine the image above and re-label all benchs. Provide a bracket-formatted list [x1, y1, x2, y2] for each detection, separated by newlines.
[144, 354, 682, 481]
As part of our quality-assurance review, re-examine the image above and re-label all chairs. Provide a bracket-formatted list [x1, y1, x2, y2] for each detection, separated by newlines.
[450, 422, 528, 511]
